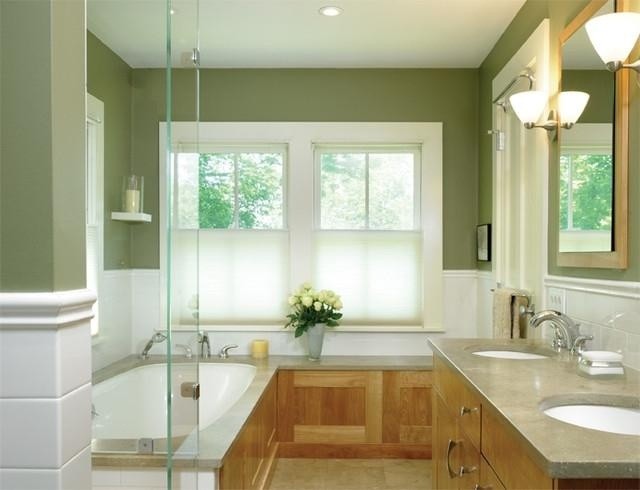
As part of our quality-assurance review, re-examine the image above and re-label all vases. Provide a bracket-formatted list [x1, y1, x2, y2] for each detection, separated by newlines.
[304, 323, 327, 361]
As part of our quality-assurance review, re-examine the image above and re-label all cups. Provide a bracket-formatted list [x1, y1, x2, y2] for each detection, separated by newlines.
[120, 174, 144, 212]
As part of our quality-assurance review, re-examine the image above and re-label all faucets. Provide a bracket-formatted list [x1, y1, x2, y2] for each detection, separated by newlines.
[197, 331, 210, 360]
[526, 308, 586, 351]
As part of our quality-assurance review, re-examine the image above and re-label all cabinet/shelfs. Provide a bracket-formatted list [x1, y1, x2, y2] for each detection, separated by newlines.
[480, 405, 553, 489]
[432, 355, 480, 488]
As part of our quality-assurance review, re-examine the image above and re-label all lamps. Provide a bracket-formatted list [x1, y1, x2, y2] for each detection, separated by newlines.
[508, 90, 558, 141]
[584, 12, 640, 72]
[558, 90, 589, 129]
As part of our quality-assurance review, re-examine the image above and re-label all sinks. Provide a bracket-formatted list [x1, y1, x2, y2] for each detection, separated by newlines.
[538, 391, 640, 435]
[470, 349, 550, 359]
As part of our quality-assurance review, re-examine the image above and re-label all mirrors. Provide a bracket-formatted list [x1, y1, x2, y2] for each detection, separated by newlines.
[556, 0, 629, 269]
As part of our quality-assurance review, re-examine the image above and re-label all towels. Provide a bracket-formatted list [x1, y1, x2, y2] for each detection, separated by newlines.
[492, 287, 531, 340]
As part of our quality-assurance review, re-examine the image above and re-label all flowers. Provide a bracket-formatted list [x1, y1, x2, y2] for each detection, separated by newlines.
[283, 284, 344, 338]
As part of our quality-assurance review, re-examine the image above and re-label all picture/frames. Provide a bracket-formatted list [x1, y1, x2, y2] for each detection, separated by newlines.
[474, 224, 492, 263]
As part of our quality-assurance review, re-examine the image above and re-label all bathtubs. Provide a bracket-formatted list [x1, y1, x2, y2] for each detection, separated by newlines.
[91, 362, 258, 438]
